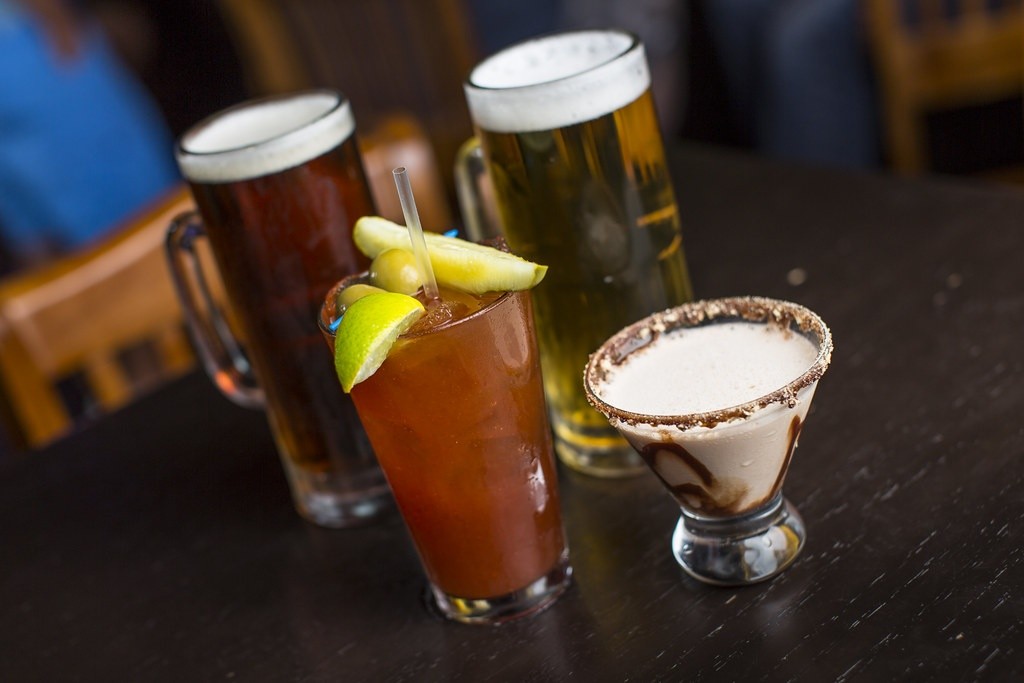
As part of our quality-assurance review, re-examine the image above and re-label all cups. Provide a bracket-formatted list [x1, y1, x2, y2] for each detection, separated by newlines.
[452, 24, 695, 476]
[583, 297, 832, 585]
[161, 91, 397, 528]
[320, 242, 572, 624]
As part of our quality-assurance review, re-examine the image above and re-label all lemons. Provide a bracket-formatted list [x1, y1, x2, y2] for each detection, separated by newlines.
[331, 214, 547, 397]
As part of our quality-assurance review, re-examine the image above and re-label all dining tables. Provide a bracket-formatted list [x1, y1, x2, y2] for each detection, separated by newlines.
[0, 142, 1023, 682]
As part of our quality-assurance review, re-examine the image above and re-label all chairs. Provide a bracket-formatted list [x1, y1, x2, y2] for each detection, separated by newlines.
[0, 112, 447, 450]
